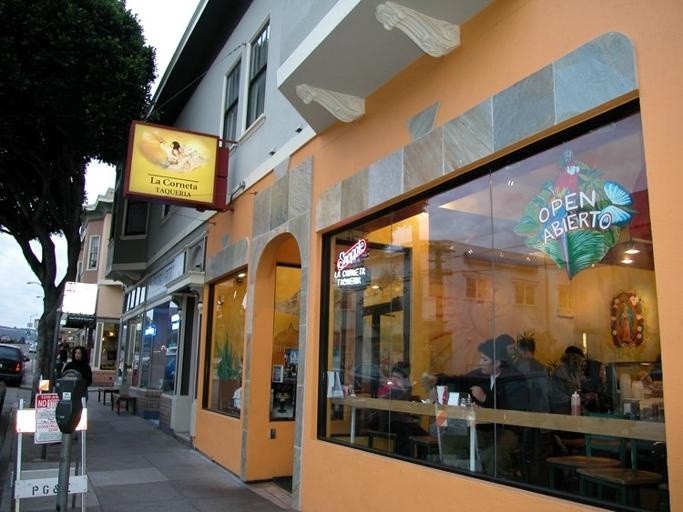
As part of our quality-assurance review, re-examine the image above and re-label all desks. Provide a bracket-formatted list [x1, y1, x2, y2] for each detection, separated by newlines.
[272, 378, 296, 408]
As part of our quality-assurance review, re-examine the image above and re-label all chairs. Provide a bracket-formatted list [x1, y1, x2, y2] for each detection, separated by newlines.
[361, 395, 439, 459]
[541, 362, 670, 512]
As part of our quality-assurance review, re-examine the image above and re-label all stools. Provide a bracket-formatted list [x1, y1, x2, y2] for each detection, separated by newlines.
[111, 393, 137, 415]
[98, 387, 120, 405]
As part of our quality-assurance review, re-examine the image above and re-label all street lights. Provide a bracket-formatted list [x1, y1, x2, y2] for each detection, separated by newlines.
[27, 281, 41, 285]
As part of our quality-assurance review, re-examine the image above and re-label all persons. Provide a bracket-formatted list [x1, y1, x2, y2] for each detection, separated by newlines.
[646, 353, 662, 382]
[160, 136, 180, 168]
[61, 346, 92, 402]
[377, 361, 431, 460]
[422, 335, 614, 477]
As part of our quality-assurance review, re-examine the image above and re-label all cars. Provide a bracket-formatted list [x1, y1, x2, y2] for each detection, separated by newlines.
[0, 344, 26, 388]
[28, 346, 36, 353]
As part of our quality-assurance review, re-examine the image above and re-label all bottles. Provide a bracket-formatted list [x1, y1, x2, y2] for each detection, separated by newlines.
[571, 391, 582, 416]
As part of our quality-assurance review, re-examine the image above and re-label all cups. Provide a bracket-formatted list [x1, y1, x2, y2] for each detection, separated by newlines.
[436, 386, 449, 405]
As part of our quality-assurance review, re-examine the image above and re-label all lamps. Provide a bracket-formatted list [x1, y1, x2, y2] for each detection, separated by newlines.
[621, 241, 640, 264]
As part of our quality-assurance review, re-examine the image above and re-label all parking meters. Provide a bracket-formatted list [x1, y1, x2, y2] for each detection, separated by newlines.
[55, 369, 86, 512]
